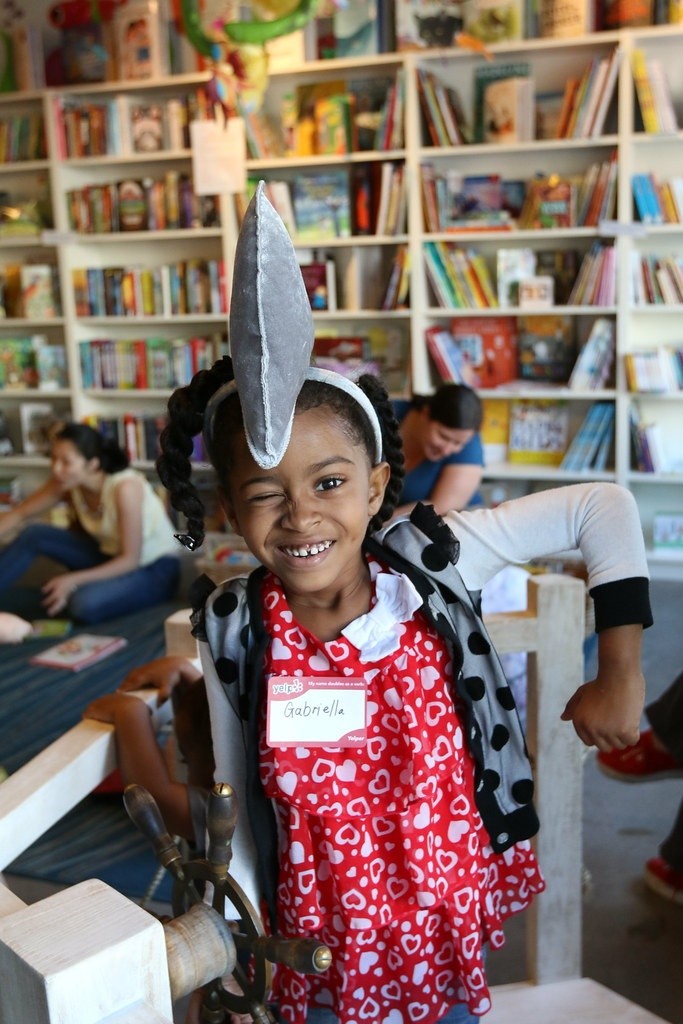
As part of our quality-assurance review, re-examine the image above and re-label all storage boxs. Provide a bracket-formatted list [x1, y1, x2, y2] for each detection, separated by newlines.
[334, 0, 394, 60]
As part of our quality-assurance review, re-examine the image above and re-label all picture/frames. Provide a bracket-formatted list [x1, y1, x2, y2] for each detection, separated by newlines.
[426, 324, 480, 390]
[19, 263, 55, 318]
[19, 401, 56, 456]
[519, 275, 556, 309]
[495, 247, 538, 309]
[34, 345, 69, 389]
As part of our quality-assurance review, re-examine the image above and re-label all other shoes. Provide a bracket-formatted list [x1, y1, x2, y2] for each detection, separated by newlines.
[595, 730, 683, 781]
[644, 857, 683, 905]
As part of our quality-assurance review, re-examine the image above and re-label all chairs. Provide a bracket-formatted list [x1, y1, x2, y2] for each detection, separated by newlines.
[168, 570, 673, 1024]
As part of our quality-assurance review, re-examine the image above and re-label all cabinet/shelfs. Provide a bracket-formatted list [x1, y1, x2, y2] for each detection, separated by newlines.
[0, 0, 683, 563]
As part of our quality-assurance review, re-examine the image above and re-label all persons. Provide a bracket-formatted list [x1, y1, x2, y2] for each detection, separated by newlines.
[596, 671, 683, 908]
[379, 383, 486, 526]
[84, 655, 216, 868]
[0, 423, 186, 625]
[204, 365, 653, 1024]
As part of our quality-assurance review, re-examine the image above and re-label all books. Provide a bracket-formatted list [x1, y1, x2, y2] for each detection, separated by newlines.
[0, 0, 683, 91]
[417, 47, 680, 147]
[323, 260, 339, 311]
[19, 264, 57, 318]
[423, 315, 683, 478]
[65, 170, 220, 233]
[71, 255, 228, 317]
[631, 171, 683, 227]
[244, 155, 620, 238]
[1, 330, 228, 464]
[0, 111, 44, 162]
[421, 240, 620, 307]
[638, 250, 683, 305]
[56, 78, 406, 160]
[377, 243, 411, 310]
